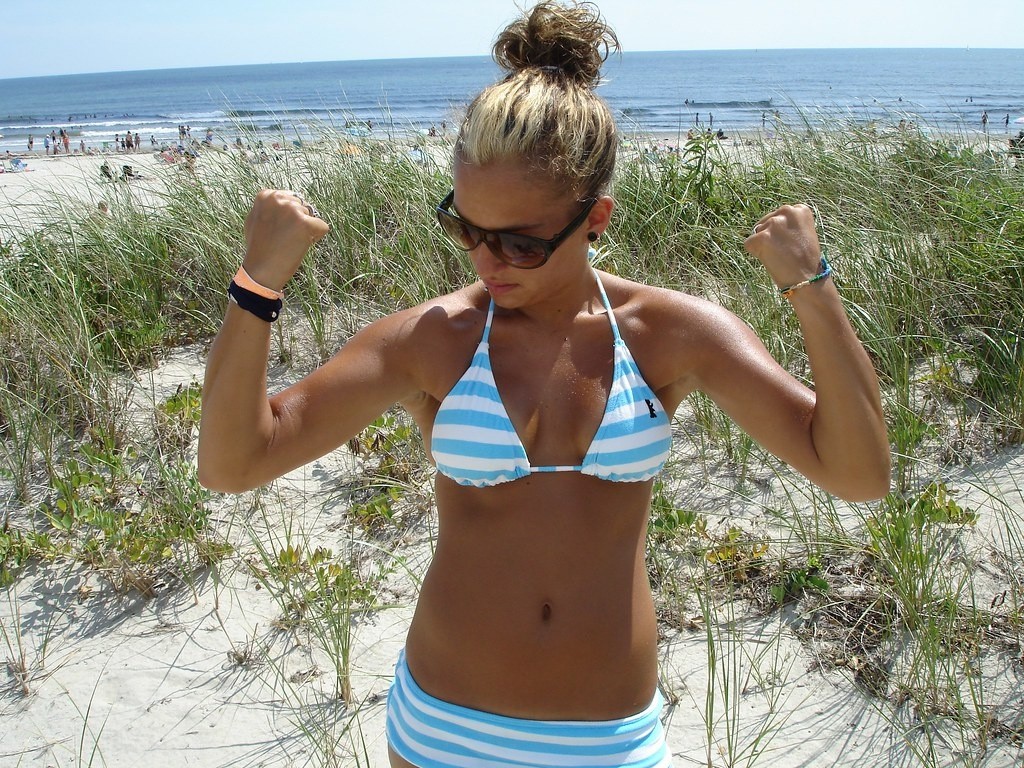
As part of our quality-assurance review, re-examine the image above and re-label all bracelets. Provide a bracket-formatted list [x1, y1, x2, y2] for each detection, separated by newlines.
[224, 263, 286, 322]
[778, 258, 832, 299]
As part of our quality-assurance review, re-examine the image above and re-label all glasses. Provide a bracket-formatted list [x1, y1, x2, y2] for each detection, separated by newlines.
[435, 189, 601, 269]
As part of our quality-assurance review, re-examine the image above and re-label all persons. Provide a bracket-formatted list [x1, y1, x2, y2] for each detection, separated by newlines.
[89, 199, 111, 227]
[0, 85, 1024, 183]
[197, 0, 892, 768]
